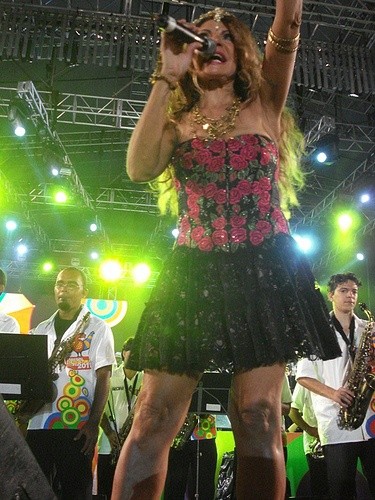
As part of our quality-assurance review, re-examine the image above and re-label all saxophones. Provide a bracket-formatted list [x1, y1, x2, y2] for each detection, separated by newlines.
[338, 298, 375, 432]
[7, 308, 91, 418]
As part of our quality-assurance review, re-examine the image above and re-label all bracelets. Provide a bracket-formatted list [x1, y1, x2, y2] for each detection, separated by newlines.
[148, 70, 177, 91]
[267, 26, 301, 55]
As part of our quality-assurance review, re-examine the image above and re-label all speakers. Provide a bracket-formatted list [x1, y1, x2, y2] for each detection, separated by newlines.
[0, 397, 58, 500]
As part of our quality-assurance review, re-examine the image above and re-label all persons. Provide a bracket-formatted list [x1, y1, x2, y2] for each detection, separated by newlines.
[1, 268, 20, 332]
[96, 335, 327, 500]
[26, 268, 116, 499]
[296, 270, 375, 500]
[111, 0, 344, 500]
[0, 399, 56, 499]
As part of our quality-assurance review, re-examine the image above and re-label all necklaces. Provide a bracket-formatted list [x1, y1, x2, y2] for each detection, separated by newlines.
[191, 100, 239, 141]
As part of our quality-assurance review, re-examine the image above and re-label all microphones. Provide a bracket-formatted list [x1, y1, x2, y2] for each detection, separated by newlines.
[158, 15, 216, 58]
